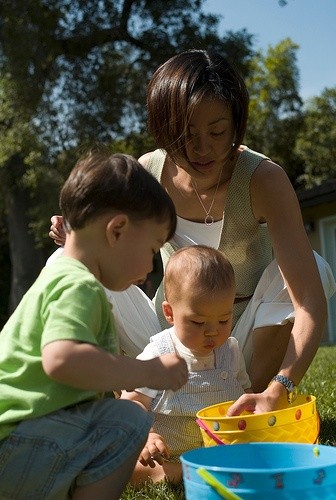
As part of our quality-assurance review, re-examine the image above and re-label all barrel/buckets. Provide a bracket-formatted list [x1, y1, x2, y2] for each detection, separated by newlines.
[180, 442, 336, 500]
[196, 394, 320, 450]
